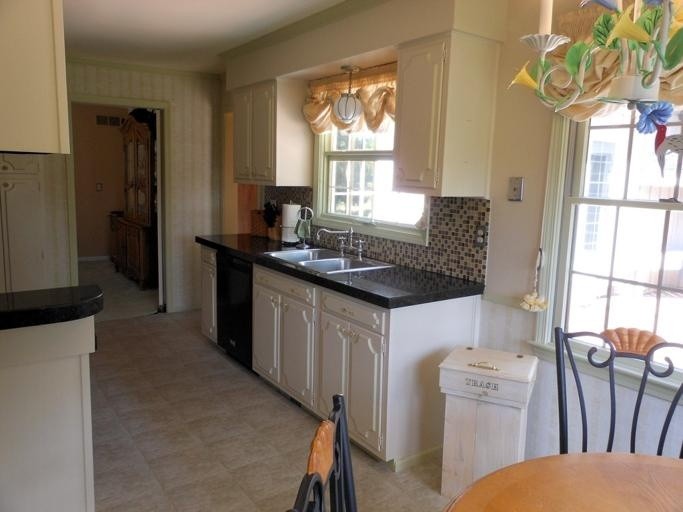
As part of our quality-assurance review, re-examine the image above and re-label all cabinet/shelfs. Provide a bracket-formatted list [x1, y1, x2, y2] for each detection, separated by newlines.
[392, 30, 501, 198]
[317, 287, 481, 462]
[231, 79, 312, 187]
[118, 113, 158, 228]
[115, 216, 158, 290]
[199, 245, 216, 344]
[252, 264, 319, 412]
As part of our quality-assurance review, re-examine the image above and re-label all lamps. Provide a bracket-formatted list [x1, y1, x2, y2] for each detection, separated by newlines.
[506, 0, 683, 135]
[332, 64, 363, 126]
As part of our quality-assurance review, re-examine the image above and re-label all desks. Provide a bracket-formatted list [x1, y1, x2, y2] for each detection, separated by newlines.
[436, 342, 540, 494]
[0, 281, 106, 511]
[444, 452, 683, 512]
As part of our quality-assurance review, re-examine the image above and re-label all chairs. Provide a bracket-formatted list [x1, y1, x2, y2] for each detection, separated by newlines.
[555, 325, 683, 458]
[287, 392, 358, 512]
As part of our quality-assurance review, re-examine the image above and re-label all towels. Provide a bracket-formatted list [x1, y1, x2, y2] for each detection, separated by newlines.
[294, 219, 311, 239]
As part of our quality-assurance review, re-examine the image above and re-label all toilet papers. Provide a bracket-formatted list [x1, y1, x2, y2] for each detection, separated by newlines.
[281, 204, 301, 243]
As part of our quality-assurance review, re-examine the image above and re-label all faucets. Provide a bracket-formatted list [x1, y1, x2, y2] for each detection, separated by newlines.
[313, 227, 353, 248]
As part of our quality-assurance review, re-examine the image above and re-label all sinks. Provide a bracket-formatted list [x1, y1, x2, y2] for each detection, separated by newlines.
[263, 248, 349, 262]
[297, 255, 401, 274]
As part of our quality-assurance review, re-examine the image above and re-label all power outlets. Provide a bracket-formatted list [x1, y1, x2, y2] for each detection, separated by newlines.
[506, 176, 525, 202]
